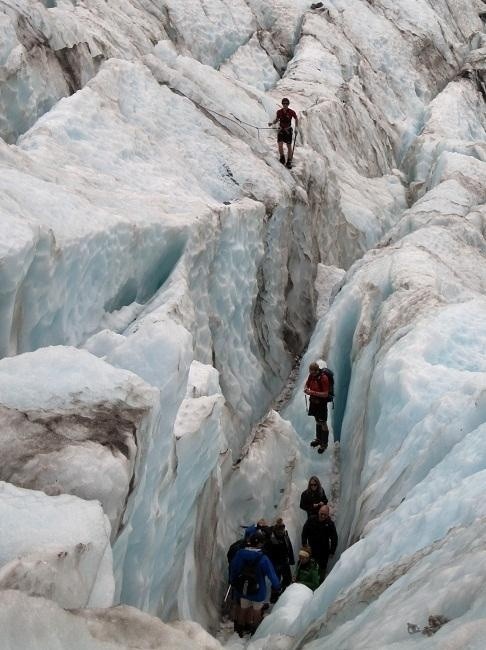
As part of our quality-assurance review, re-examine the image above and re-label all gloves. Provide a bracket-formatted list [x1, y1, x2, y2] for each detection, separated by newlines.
[270, 596, 278, 603]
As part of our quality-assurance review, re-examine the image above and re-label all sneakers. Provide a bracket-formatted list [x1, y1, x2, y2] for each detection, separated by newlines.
[311, 439, 321, 446]
[318, 444, 328, 453]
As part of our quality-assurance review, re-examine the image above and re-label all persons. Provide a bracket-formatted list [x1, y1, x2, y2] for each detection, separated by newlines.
[300, 476, 328, 516]
[292, 545, 321, 589]
[302, 362, 331, 454]
[224, 519, 290, 638]
[268, 97, 298, 168]
[302, 505, 338, 569]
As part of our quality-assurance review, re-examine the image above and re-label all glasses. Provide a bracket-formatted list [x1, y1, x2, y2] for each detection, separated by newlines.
[311, 484, 316, 486]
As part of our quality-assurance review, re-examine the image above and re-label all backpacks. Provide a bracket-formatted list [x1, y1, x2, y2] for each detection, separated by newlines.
[318, 368, 334, 402]
[233, 554, 264, 596]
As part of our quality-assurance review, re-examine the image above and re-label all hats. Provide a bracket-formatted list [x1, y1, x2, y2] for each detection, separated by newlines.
[245, 526, 266, 545]
[299, 545, 311, 558]
